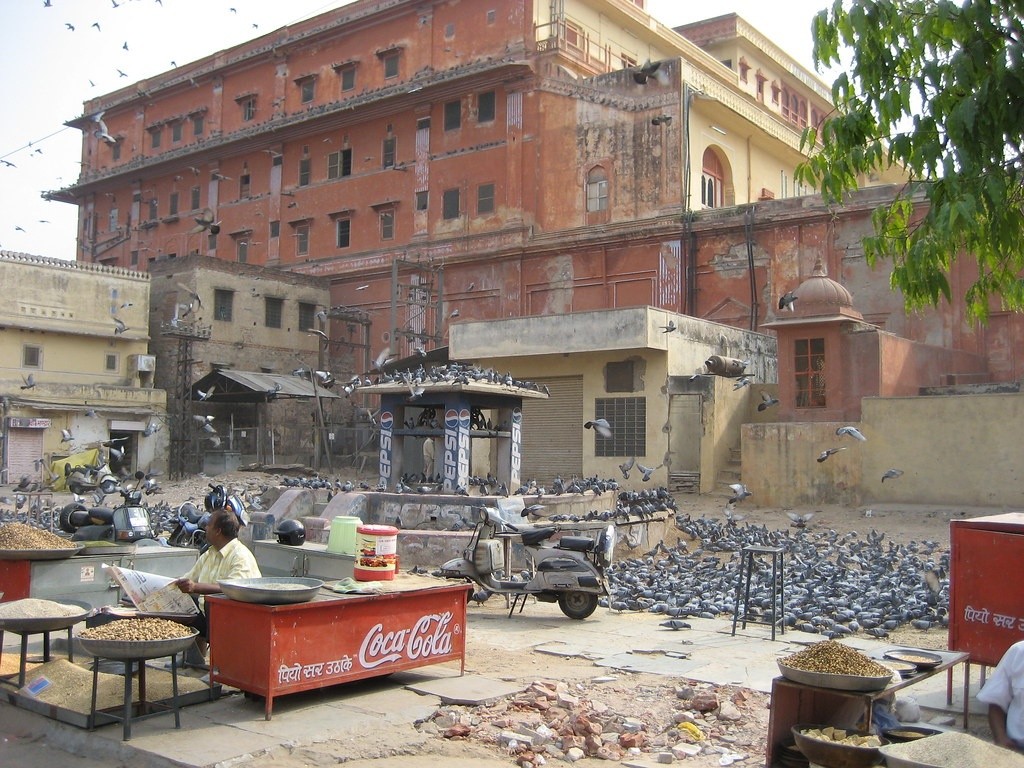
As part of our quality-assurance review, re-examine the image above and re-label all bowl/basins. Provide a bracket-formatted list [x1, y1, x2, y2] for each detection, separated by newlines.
[878, 745, 1024, 768]
[778, 653, 893, 691]
[217, 577, 325, 604]
[881, 726, 944, 743]
[75, 625, 200, 658]
[790, 723, 892, 768]
[0, 598, 94, 632]
[0, 541, 85, 559]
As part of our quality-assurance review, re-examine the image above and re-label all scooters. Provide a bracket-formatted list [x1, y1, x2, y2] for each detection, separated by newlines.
[428, 504, 615, 619]
[67, 455, 118, 495]
[69, 470, 164, 543]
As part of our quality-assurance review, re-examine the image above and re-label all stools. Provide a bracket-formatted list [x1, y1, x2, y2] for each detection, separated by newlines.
[0, 625, 74, 690]
[90, 653, 180, 740]
[733, 545, 786, 640]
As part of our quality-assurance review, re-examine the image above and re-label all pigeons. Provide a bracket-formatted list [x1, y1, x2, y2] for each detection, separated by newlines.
[0, 0, 967, 641]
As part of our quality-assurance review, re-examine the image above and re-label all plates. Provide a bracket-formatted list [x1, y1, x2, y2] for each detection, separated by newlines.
[872, 659, 917, 677]
[886, 648, 942, 670]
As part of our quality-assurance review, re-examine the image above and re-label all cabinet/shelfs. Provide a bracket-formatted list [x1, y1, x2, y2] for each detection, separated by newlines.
[766, 648, 971, 768]
[202, 580, 473, 721]
[1, 547, 199, 621]
[947, 513, 1024, 705]
[252, 538, 357, 582]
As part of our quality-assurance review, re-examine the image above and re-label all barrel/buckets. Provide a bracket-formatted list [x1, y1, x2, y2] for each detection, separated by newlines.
[354, 524, 400, 581]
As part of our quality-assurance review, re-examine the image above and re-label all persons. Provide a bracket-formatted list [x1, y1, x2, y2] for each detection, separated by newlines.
[988, 641, 1024, 753]
[165, 508, 263, 668]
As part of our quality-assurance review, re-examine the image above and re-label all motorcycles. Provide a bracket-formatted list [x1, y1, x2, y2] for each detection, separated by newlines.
[163, 482, 251, 555]
